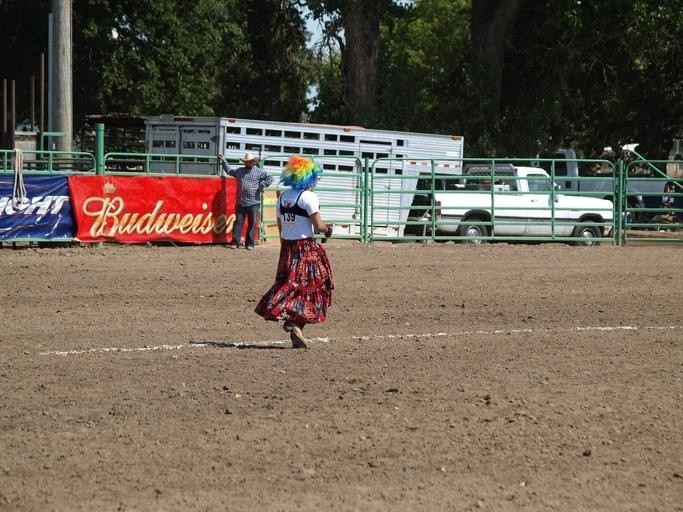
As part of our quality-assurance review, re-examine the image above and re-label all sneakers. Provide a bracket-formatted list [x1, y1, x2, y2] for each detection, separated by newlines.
[282, 320, 308, 348]
[230, 245, 253, 250]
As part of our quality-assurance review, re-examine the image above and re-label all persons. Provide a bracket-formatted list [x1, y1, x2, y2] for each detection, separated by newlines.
[253, 155, 334, 350]
[217, 152, 274, 250]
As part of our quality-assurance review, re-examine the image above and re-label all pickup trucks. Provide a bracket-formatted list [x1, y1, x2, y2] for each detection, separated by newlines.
[508, 147, 676, 232]
[410, 163, 616, 247]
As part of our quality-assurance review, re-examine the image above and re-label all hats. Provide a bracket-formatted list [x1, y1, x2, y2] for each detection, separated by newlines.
[240, 152, 259, 165]
